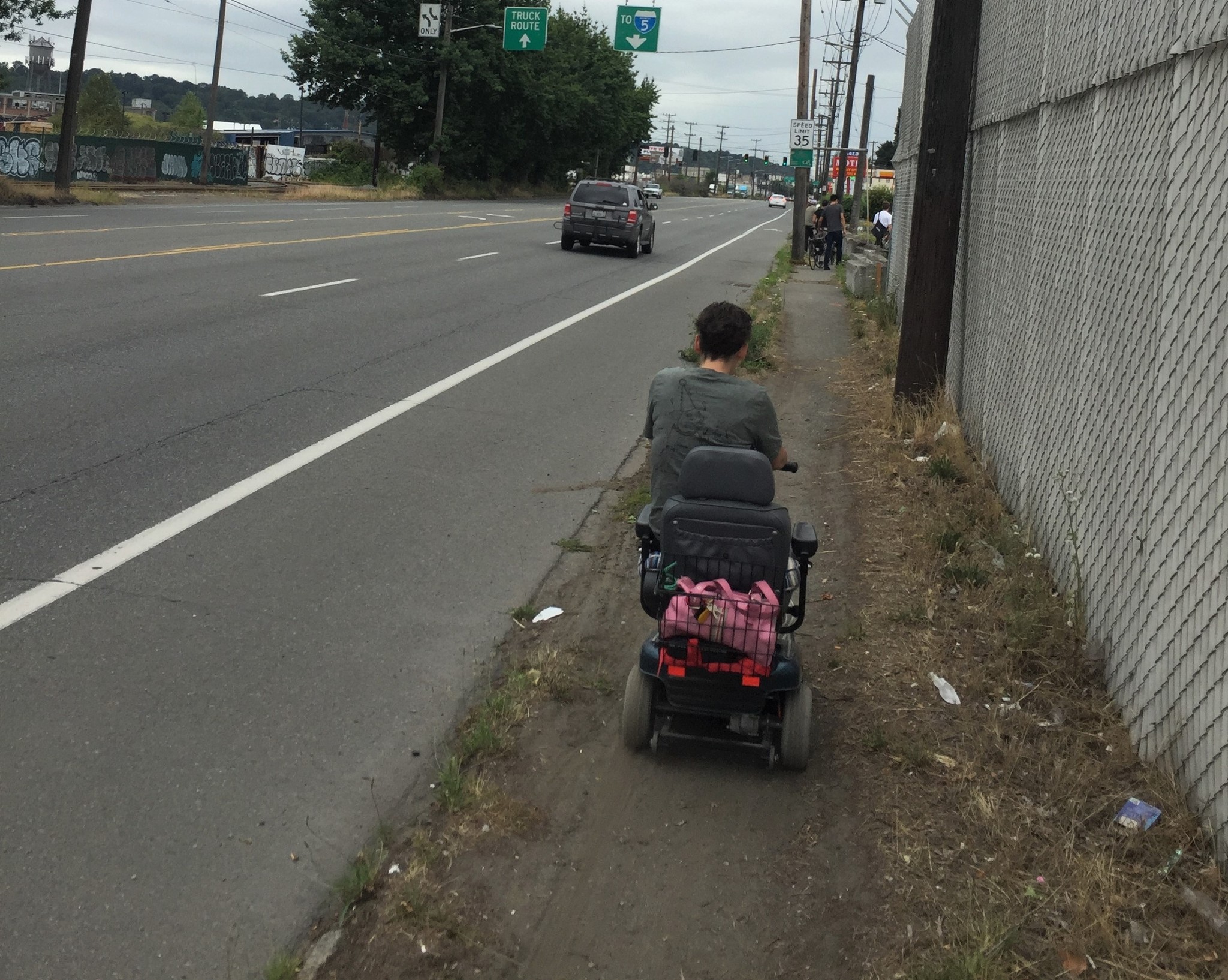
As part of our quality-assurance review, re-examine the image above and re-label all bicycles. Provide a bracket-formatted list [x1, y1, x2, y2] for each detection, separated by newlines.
[807, 226, 829, 270]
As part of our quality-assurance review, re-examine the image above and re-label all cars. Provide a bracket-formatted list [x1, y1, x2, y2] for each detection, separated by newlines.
[768, 194, 787, 209]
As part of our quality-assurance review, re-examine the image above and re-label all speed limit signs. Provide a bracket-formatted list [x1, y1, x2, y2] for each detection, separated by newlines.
[789, 119, 815, 150]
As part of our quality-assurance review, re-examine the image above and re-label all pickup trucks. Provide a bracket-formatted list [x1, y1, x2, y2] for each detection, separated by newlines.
[642, 183, 663, 199]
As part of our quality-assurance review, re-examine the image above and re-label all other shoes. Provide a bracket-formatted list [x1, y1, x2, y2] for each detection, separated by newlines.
[824, 267, 830, 270]
[836, 261, 841, 266]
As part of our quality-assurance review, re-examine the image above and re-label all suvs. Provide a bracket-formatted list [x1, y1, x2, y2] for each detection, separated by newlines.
[561, 175, 658, 259]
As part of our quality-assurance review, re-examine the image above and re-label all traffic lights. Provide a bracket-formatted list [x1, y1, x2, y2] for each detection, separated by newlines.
[744, 154, 749, 163]
[783, 157, 788, 166]
[764, 156, 769, 165]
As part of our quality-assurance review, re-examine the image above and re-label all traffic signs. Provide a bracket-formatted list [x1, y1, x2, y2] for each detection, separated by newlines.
[613, 5, 662, 53]
[502, 6, 548, 51]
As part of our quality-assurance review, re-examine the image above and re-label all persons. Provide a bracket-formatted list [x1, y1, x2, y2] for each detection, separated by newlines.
[872, 200, 892, 247]
[804, 194, 846, 270]
[642, 301, 787, 555]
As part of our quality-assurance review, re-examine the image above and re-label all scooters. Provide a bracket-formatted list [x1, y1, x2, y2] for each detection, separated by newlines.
[622, 446, 819, 772]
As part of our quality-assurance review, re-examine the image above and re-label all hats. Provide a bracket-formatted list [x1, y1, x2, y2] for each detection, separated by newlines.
[811, 200, 817, 204]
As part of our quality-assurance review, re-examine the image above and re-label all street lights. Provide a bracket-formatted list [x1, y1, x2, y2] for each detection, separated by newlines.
[298, 85, 306, 148]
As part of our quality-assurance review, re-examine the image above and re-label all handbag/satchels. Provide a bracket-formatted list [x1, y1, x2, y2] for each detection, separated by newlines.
[871, 212, 888, 239]
[660, 575, 780, 667]
